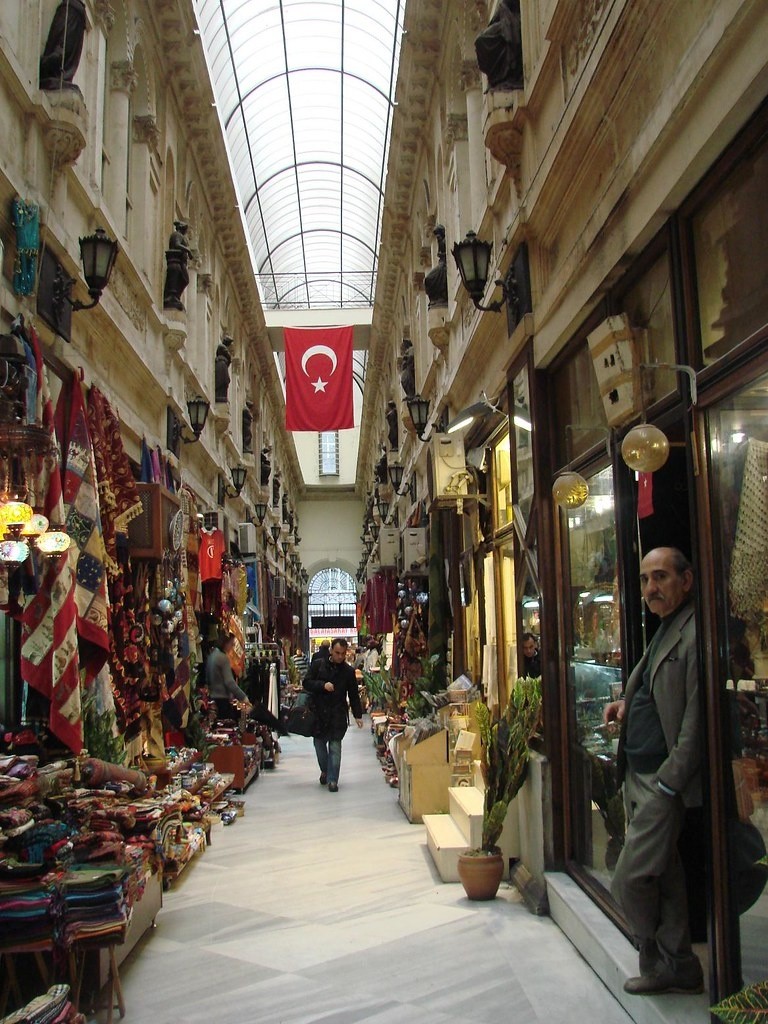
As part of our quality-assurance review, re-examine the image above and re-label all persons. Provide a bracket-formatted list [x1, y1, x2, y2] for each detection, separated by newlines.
[251, 639, 379, 686]
[362, 223, 448, 535]
[163, 221, 303, 543]
[302, 638, 363, 792]
[602, 548, 707, 995]
[726, 639, 768, 917]
[205, 632, 249, 727]
[523, 633, 542, 680]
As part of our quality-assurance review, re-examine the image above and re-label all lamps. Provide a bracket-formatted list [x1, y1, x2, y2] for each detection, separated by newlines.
[552, 424, 609, 509]
[274, 539, 309, 587]
[264, 521, 282, 550]
[246, 497, 268, 531]
[406, 394, 449, 442]
[620, 364, 673, 474]
[351, 497, 398, 583]
[388, 461, 416, 504]
[449, 229, 528, 335]
[167, 393, 211, 459]
[35, 226, 119, 344]
[218, 463, 247, 508]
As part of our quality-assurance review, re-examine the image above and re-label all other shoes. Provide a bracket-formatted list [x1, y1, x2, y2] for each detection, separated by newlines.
[320, 771, 327, 785]
[328, 780, 338, 792]
[624, 972, 704, 995]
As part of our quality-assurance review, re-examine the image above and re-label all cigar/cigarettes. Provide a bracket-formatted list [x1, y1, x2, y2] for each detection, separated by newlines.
[591, 721, 618, 730]
[750, 714, 765, 721]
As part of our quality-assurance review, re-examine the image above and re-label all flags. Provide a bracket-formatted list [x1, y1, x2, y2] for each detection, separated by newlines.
[283, 324, 354, 430]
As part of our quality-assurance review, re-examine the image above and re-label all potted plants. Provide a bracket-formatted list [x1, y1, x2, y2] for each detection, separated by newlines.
[457, 674, 544, 901]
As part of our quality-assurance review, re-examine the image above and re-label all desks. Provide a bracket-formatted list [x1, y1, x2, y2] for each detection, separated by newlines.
[163, 772, 236, 877]
[74, 842, 163, 1014]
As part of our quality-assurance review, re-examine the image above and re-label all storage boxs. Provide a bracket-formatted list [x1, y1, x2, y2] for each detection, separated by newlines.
[394, 726, 478, 825]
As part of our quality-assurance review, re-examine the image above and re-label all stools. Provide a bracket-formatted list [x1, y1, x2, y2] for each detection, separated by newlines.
[1, 932, 130, 1023]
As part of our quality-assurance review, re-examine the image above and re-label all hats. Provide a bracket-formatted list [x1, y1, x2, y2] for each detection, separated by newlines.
[321, 640, 330, 647]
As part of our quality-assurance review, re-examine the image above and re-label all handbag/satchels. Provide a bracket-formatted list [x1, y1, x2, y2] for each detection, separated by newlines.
[286, 688, 316, 737]
[404, 614, 426, 655]
